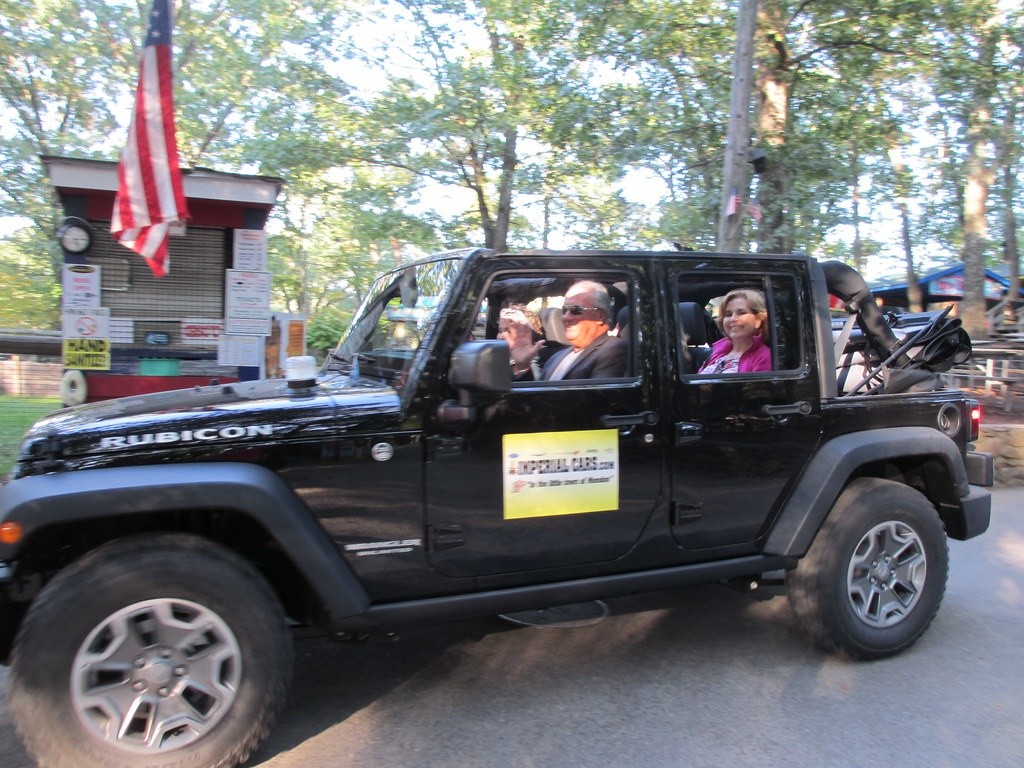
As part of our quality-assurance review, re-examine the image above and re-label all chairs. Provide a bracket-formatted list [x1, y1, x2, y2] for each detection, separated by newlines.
[679, 302, 711, 373]
[617, 305, 633, 377]
[541, 307, 566, 344]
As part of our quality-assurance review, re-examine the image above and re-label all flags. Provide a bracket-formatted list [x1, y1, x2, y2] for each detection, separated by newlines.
[725, 185, 742, 221]
[748, 192, 762, 223]
[107, 0, 190, 280]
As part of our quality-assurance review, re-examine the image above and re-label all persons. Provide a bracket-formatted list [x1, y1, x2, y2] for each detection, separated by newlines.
[508, 278, 631, 382]
[491, 300, 563, 382]
[694, 286, 772, 374]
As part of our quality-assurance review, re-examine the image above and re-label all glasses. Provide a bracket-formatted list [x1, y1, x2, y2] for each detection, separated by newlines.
[562, 305, 602, 315]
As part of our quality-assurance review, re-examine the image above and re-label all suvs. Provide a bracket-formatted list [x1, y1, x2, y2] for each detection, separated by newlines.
[0, 247, 998, 768]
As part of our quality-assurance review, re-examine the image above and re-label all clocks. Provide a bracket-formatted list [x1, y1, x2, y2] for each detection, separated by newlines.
[57, 222, 93, 256]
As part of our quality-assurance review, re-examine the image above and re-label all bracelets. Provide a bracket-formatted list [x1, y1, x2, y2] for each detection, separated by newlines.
[511, 364, 530, 375]
